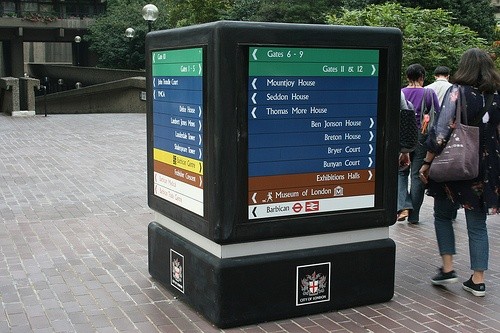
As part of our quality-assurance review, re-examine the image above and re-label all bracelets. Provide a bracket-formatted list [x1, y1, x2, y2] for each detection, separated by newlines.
[423, 158, 431, 165]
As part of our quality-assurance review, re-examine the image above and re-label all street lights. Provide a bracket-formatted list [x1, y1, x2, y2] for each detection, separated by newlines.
[75, 35, 81, 65]
[125, 28, 135, 70]
[142, 4, 159, 32]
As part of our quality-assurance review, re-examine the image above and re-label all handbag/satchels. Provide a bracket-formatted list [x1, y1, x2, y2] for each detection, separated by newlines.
[426, 85, 480, 183]
[399, 109, 418, 148]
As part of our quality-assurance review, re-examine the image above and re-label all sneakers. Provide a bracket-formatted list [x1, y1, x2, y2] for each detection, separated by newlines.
[462, 274, 486, 296]
[428, 265, 458, 285]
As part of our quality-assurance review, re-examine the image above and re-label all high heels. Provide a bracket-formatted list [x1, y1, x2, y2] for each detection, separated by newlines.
[396, 209, 409, 221]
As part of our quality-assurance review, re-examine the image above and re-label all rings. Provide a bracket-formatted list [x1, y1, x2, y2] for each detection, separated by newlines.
[419, 173, 423, 176]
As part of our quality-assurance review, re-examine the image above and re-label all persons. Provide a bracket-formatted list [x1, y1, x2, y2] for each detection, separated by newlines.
[419, 49, 500, 297]
[396, 63, 457, 224]
